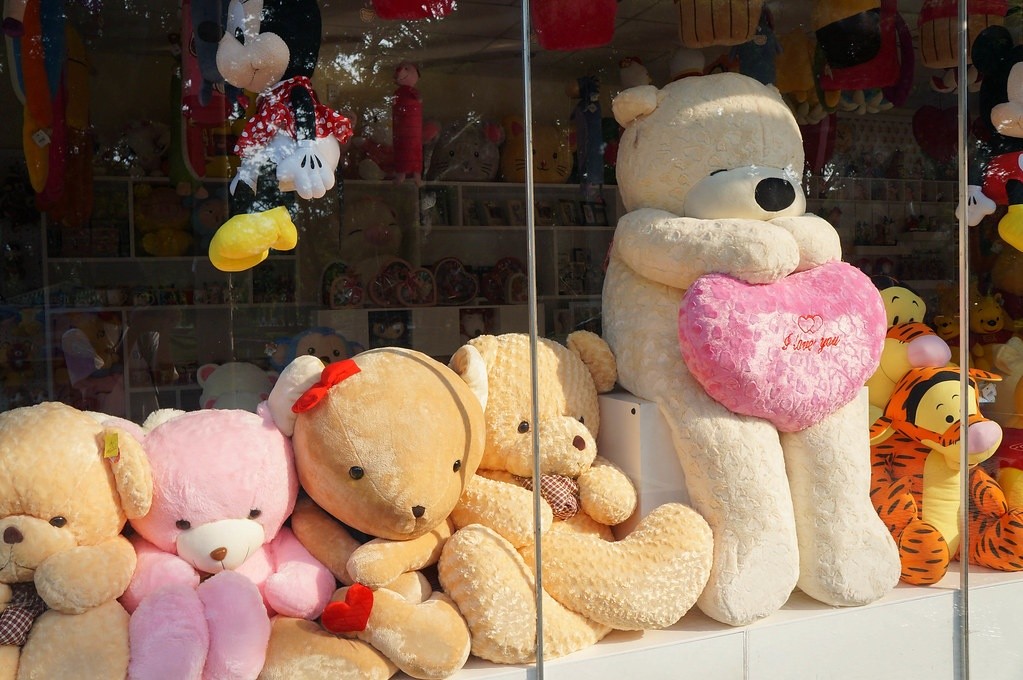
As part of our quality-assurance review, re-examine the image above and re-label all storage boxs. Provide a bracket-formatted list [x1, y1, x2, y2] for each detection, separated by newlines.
[318, 254, 527, 307]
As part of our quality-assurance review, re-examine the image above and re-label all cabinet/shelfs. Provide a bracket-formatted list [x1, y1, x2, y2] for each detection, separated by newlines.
[801, 176, 959, 321]
[41, 178, 631, 428]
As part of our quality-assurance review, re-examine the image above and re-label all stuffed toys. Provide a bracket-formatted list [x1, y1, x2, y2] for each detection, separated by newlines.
[0, 0, 1023, 680]
